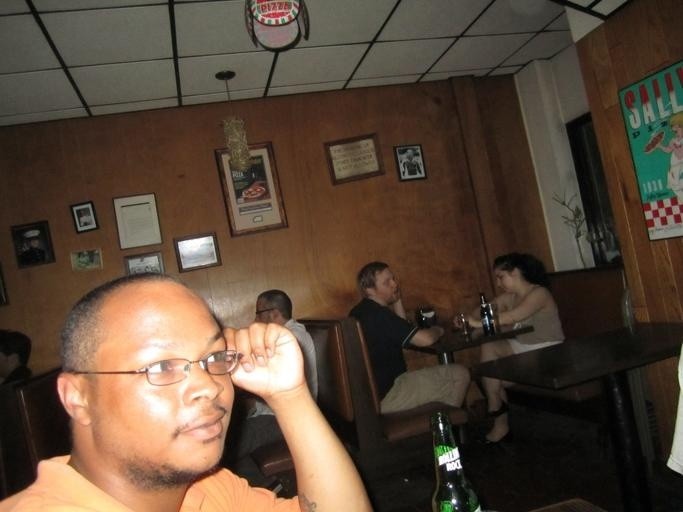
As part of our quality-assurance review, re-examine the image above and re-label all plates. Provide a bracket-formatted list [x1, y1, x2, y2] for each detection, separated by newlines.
[643, 130, 665, 154]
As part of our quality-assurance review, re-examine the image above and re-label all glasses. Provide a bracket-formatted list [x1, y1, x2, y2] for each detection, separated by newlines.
[74, 349, 243, 389]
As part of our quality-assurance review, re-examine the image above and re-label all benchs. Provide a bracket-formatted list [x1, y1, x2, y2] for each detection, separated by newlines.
[473, 263, 633, 447]
[251, 317, 468, 480]
[11, 365, 70, 482]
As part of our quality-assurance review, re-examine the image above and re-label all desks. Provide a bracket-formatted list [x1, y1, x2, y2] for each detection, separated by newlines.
[399, 318, 535, 369]
[472, 321, 683, 512]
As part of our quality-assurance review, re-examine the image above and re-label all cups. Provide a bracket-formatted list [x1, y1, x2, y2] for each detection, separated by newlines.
[455, 311, 470, 334]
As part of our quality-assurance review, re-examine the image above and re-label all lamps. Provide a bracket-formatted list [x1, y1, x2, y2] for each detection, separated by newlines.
[215, 69, 252, 173]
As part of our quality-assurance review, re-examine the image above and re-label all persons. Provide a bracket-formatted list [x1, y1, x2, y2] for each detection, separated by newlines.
[453, 253, 566, 450]
[656, 112, 683, 204]
[349, 261, 471, 415]
[402, 149, 422, 176]
[218, 290, 319, 468]
[0, 329, 32, 387]
[0, 272, 375, 512]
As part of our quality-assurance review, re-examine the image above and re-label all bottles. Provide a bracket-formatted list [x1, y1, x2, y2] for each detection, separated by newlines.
[418, 306, 427, 331]
[478, 293, 494, 332]
[428, 410, 483, 512]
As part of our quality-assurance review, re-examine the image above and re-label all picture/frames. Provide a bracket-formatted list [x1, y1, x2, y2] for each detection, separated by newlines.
[321, 133, 385, 186]
[70, 200, 99, 234]
[112, 191, 163, 250]
[124, 250, 165, 278]
[618, 59, 683, 241]
[70, 247, 104, 273]
[173, 231, 222, 272]
[215, 141, 288, 237]
[11, 219, 56, 268]
[393, 145, 427, 182]
